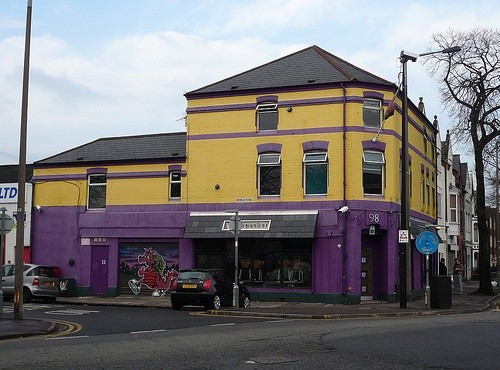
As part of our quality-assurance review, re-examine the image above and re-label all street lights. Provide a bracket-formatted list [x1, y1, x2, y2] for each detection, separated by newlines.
[399, 46, 462, 308]
[444, 128, 470, 246]
[224, 208, 240, 308]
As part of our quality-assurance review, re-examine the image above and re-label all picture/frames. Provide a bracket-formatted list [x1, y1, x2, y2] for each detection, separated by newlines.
[362, 285, 368, 293]
[362, 256, 367, 264]
[362, 271, 368, 279]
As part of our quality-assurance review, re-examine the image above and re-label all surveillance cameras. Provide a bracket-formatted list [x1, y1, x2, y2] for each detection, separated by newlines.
[36, 205, 41, 210]
[338, 205, 348, 213]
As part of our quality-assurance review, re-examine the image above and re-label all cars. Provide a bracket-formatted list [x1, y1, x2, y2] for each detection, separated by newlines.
[0, 262, 77, 304]
[169, 268, 251, 311]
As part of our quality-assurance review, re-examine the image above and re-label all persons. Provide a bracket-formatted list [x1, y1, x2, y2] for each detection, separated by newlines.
[452, 258, 463, 295]
[439, 258, 447, 275]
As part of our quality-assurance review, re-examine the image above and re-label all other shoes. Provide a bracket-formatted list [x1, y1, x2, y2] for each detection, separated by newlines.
[459, 292, 463, 294]
[452, 291, 457, 294]
